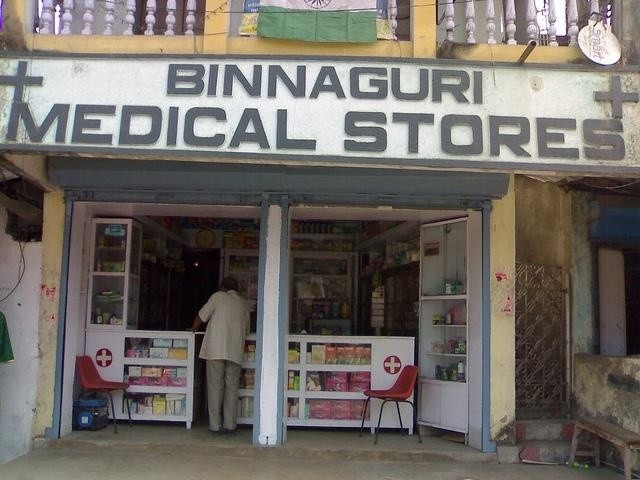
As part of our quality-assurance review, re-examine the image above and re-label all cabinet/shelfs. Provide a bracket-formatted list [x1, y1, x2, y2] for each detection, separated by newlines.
[415, 214, 469, 298]
[123, 336, 189, 422]
[417, 298, 467, 385]
[90, 225, 140, 326]
[419, 382, 470, 433]
[234, 340, 373, 428]
[219, 224, 418, 333]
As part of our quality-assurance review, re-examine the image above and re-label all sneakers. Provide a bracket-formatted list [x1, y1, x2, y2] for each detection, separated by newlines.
[207, 428, 237, 436]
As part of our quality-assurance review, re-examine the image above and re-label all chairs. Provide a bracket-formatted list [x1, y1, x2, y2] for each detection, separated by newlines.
[75, 356, 133, 433]
[359, 363, 416, 444]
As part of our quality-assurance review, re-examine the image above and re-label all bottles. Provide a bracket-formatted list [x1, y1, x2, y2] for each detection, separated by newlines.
[110, 313, 117, 325]
[456, 360, 465, 382]
[95, 307, 103, 323]
[313, 295, 350, 319]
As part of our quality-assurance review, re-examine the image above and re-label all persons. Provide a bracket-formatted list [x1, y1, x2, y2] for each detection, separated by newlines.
[185, 275, 251, 435]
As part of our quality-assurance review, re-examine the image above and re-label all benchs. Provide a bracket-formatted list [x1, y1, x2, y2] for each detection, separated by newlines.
[569, 411, 639, 480]
